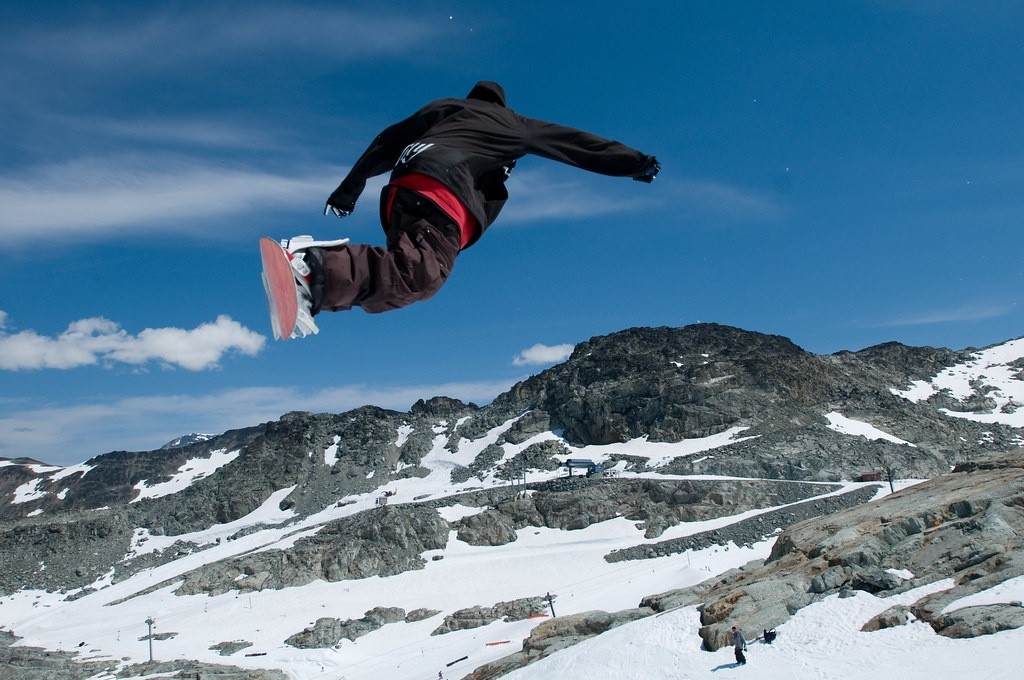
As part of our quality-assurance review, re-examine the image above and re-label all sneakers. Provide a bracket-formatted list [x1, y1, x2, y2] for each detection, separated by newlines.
[285, 250, 320, 340]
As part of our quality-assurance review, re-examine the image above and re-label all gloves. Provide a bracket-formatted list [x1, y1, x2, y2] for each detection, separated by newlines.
[323, 204, 351, 219]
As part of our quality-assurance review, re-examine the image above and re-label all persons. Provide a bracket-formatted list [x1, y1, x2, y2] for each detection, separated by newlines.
[262, 81, 661, 341]
[731, 626, 747, 665]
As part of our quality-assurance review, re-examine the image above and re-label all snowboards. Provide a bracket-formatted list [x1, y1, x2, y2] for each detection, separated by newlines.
[259, 235, 298, 341]
